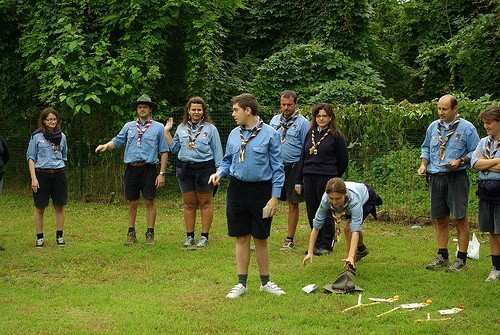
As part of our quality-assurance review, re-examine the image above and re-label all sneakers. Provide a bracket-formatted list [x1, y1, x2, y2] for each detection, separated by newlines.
[145, 231, 155, 245]
[197, 236, 208, 247]
[225, 237, 370, 300]
[425, 257, 446, 269]
[484, 267, 500, 282]
[449, 260, 466, 273]
[124, 231, 137, 245]
[36, 237, 44, 249]
[57, 235, 66, 246]
[182, 236, 194, 247]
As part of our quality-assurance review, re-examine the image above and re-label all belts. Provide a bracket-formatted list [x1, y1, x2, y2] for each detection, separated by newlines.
[35, 168, 64, 174]
[132, 162, 145, 166]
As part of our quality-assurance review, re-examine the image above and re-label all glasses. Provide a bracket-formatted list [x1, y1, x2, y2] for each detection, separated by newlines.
[42, 118, 57, 122]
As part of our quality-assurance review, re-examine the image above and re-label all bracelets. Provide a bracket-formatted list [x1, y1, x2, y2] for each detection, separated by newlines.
[160, 171, 165, 176]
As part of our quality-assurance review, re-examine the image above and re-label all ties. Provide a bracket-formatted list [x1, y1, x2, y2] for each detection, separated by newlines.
[187, 120, 204, 149]
[239, 118, 263, 163]
[48, 140, 58, 154]
[330, 194, 348, 240]
[482, 135, 500, 175]
[280, 110, 300, 143]
[437, 114, 460, 161]
[136, 117, 152, 147]
[309, 128, 332, 154]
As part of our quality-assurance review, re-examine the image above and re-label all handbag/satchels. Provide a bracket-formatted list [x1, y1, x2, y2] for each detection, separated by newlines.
[455, 233, 479, 260]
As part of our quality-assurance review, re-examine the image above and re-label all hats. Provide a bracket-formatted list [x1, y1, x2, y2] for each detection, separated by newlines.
[134, 94, 159, 111]
[323, 272, 365, 293]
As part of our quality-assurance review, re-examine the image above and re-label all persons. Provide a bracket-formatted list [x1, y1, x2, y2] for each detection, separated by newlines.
[303, 177, 383, 269]
[417, 94, 480, 273]
[26, 107, 68, 248]
[294, 104, 349, 255]
[95, 94, 169, 246]
[164, 97, 224, 248]
[471, 106, 500, 282]
[251, 90, 311, 251]
[208, 93, 286, 299]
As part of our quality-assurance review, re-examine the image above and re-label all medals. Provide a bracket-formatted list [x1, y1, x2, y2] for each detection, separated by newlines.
[188, 142, 194, 149]
[281, 137, 286, 143]
